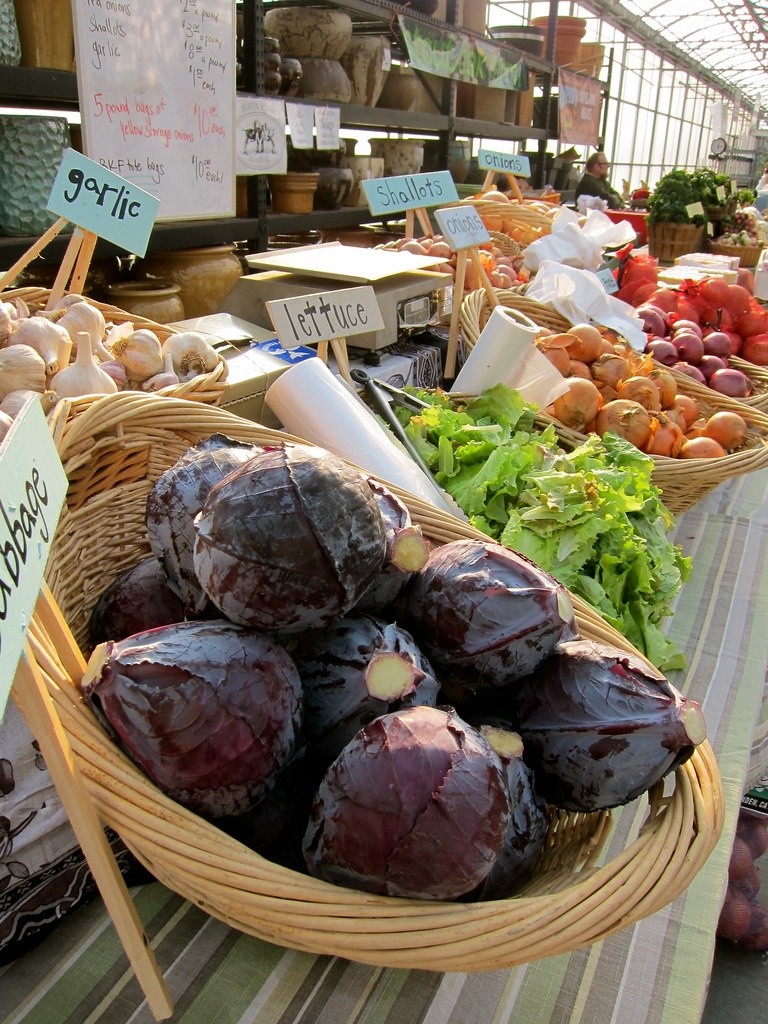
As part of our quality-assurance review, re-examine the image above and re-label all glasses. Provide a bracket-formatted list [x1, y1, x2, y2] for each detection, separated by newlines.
[598, 162, 613, 168]
[764, 169, 768, 173]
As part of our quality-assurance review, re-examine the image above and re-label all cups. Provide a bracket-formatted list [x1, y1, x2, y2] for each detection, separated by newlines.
[341, 155, 385, 207]
[271, 172, 319, 213]
[339, 34, 391, 108]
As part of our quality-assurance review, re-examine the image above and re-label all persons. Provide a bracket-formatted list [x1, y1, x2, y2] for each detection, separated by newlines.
[575, 152, 625, 211]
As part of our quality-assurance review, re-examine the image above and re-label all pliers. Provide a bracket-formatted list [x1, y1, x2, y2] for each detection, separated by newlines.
[368, 378, 470, 526]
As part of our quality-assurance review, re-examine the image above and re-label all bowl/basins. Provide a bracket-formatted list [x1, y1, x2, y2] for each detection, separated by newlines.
[421, 140, 471, 184]
[295, 58, 351, 104]
[313, 168, 353, 210]
[604, 209, 649, 245]
[368, 138, 426, 177]
[486, 26, 545, 57]
[263, 6, 352, 58]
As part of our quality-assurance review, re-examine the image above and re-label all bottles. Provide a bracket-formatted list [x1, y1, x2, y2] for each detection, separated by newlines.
[104, 283, 185, 324]
[146, 242, 242, 318]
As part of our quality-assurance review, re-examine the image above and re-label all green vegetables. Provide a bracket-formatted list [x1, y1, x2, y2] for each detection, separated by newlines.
[645, 168, 758, 229]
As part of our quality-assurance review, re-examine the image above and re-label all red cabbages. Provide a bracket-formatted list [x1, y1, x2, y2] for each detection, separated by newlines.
[77, 433, 707, 905]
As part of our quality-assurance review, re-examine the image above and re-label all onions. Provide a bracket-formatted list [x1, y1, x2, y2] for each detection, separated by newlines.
[394, 191, 592, 288]
[534, 259, 768, 459]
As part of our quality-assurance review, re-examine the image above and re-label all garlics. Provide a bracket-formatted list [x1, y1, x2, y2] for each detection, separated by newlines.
[0, 293, 221, 445]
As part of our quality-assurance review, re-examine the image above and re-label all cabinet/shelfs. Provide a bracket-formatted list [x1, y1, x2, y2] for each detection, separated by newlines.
[0, 0, 609, 260]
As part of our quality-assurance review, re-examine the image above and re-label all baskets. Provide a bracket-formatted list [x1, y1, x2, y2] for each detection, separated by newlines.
[439, 197, 559, 266]
[459, 287, 768, 517]
[22, 389, 725, 972]
[706, 237, 762, 266]
[0, 286, 229, 515]
[726, 354, 768, 415]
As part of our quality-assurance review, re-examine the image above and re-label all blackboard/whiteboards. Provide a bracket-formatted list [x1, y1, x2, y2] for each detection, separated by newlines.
[71, 1, 237, 225]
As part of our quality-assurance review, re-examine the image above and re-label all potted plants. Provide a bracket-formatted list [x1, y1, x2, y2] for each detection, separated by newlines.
[646, 166, 755, 263]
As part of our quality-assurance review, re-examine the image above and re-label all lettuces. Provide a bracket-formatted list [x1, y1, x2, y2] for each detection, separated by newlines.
[387, 383, 694, 674]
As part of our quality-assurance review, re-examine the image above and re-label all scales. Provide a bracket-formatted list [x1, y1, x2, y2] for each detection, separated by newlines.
[217, 240, 454, 367]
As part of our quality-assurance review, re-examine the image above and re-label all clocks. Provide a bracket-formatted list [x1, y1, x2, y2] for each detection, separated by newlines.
[710, 137, 727, 154]
